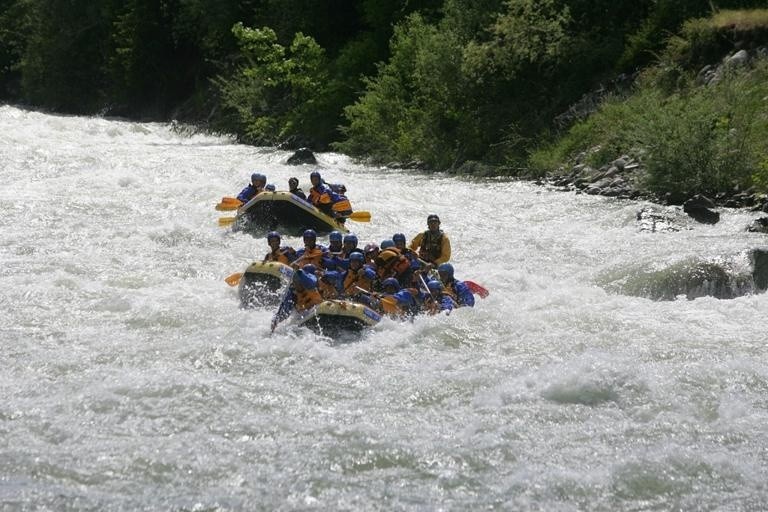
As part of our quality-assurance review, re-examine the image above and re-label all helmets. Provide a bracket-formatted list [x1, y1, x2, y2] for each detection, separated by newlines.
[266, 184, 275, 189]
[311, 172, 320, 183]
[267, 231, 281, 244]
[334, 184, 346, 193]
[289, 178, 298, 189]
[251, 173, 261, 183]
[427, 215, 441, 225]
[295, 229, 453, 296]
[260, 175, 266, 187]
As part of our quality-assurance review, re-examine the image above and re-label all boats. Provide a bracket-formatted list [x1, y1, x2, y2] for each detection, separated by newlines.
[230, 190, 346, 241]
[297, 300, 383, 340]
[237, 260, 295, 308]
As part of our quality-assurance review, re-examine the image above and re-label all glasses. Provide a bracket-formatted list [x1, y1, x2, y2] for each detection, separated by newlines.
[429, 221, 438, 224]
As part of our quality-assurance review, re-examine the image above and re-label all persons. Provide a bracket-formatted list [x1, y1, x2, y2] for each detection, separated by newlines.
[237, 172, 475, 318]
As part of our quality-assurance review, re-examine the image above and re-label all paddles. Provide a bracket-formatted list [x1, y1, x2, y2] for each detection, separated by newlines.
[338, 212, 371, 222]
[216, 196, 243, 211]
[327, 200, 350, 213]
[355, 285, 403, 314]
[218, 218, 236, 226]
[225, 272, 243, 287]
[317, 192, 331, 204]
